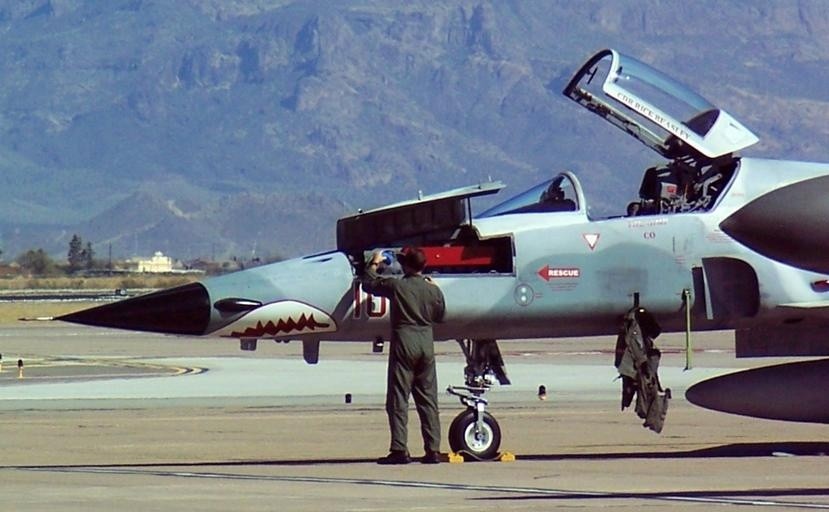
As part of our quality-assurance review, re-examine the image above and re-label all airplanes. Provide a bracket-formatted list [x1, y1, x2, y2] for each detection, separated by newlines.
[16, 47, 829, 462]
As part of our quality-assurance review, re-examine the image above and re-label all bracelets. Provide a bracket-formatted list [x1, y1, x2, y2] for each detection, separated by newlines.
[371, 262, 378, 266]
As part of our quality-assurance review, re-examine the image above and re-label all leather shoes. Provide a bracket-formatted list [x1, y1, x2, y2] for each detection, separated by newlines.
[378, 450, 409, 462]
[418, 452, 442, 465]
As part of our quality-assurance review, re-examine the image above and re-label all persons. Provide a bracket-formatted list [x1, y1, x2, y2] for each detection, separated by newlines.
[358, 245, 446, 464]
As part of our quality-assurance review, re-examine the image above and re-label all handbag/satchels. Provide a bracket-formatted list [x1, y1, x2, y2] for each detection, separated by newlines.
[365, 248, 403, 274]
